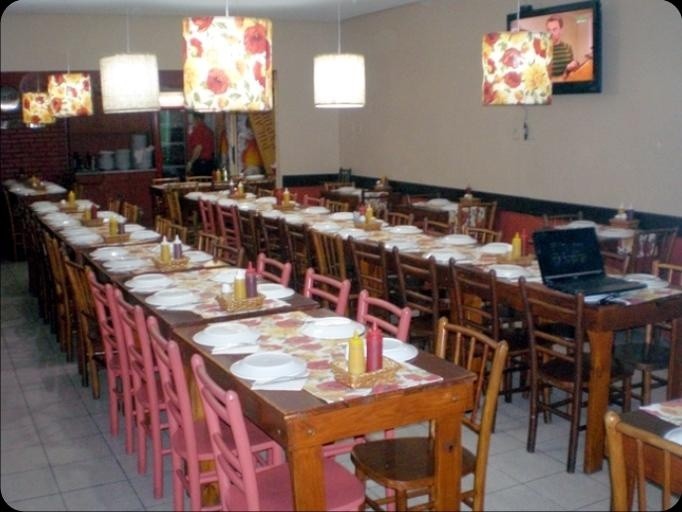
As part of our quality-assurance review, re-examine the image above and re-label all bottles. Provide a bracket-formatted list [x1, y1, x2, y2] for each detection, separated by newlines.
[245, 261, 256, 298]
[347, 329, 366, 374]
[366, 321, 383, 372]
[233, 270, 246, 300]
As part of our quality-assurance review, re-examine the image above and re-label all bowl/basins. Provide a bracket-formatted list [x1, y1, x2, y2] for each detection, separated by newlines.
[256, 282, 296, 301]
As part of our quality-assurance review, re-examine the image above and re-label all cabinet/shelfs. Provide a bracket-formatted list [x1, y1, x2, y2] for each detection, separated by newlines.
[61, 86, 164, 232]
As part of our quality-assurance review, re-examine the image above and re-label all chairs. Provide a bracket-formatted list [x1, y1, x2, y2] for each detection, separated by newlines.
[255, 252, 510, 512]
[109, 188, 257, 265]
[258, 212, 397, 310]
[304, 181, 503, 244]
[86, 265, 366, 511]
[520, 207, 681, 475]
[22, 199, 107, 399]
[607, 409, 682, 511]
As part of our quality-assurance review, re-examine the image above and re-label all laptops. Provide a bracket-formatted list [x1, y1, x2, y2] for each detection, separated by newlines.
[533, 227, 647, 296]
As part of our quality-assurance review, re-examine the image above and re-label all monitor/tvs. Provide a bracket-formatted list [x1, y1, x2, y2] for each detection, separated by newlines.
[507, 0, 602, 93]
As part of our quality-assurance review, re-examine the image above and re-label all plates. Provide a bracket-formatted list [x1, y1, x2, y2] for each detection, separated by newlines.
[147, 289, 204, 304]
[192, 322, 259, 346]
[207, 268, 246, 282]
[302, 314, 362, 338]
[345, 336, 416, 364]
[125, 273, 172, 291]
[181, 175, 669, 295]
[98, 134, 155, 170]
[231, 350, 306, 380]
[5, 173, 216, 273]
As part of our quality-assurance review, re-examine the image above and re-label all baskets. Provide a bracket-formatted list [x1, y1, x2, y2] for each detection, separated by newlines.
[150, 254, 190, 272]
[27, 184, 105, 226]
[459, 196, 480, 207]
[496, 252, 534, 268]
[609, 219, 640, 230]
[228, 194, 247, 201]
[100, 229, 131, 245]
[355, 218, 383, 232]
[215, 291, 265, 312]
[272, 203, 295, 211]
[329, 353, 400, 391]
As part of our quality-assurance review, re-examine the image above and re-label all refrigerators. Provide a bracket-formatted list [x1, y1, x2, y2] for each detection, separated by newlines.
[154, 91, 238, 179]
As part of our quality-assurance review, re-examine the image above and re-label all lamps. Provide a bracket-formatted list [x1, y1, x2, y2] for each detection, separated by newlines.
[478, 1, 555, 110]
[21, 69, 57, 128]
[97, 7, 163, 114]
[312, 2, 367, 111]
[44, 30, 94, 119]
[180, 1, 275, 114]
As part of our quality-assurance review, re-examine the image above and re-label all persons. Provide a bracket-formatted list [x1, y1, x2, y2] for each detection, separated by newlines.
[183, 112, 217, 224]
[540, 14, 577, 75]
